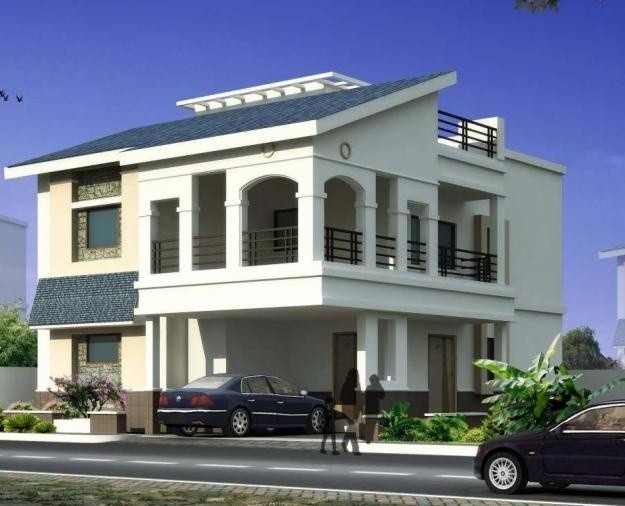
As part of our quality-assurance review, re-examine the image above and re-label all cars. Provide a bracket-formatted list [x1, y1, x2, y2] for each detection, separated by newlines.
[473, 399, 625, 494]
[157, 371, 332, 437]
